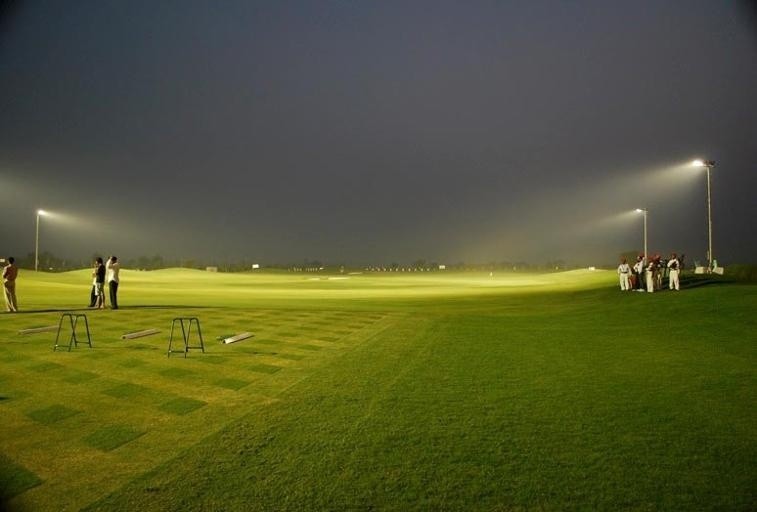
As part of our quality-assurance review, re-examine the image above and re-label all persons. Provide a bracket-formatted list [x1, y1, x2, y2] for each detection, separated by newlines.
[655, 253, 667, 290]
[105, 256, 120, 310]
[1, 256, 18, 312]
[617, 256, 633, 292]
[94, 256, 106, 309]
[643, 256, 657, 293]
[633, 255, 645, 292]
[87, 261, 99, 307]
[667, 252, 681, 292]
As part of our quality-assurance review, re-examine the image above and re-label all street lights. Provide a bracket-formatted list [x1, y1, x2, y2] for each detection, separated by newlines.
[693, 158, 716, 268]
[33, 210, 43, 274]
[636, 207, 648, 268]
